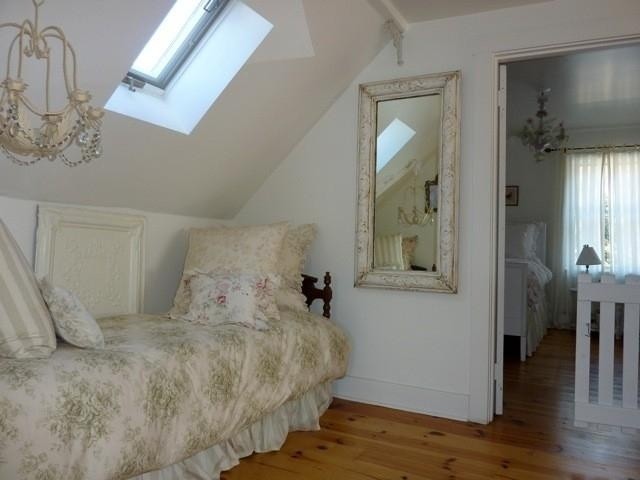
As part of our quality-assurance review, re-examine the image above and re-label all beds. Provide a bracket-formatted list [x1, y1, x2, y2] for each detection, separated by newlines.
[504, 220, 551, 362]
[0, 271, 351, 480]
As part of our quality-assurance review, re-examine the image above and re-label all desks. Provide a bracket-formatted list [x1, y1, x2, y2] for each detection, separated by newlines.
[569, 288, 622, 341]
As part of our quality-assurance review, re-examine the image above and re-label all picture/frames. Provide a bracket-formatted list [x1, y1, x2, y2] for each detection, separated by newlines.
[506, 185, 519, 206]
[424, 181, 438, 213]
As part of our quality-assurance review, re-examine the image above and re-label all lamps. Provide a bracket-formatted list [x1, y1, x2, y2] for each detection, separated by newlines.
[396, 160, 433, 227]
[520, 86, 570, 164]
[0, 0, 106, 169]
[575, 244, 602, 283]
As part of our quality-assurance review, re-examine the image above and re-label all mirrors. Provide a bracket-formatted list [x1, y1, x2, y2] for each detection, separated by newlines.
[352, 69, 462, 294]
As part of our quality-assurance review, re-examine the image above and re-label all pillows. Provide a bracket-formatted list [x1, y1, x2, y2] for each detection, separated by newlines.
[38, 278, 105, 351]
[1, 220, 57, 359]
[164, 220, 318, 333]
[505, 224, 537, 259]
[373, 233, 418, 270]
